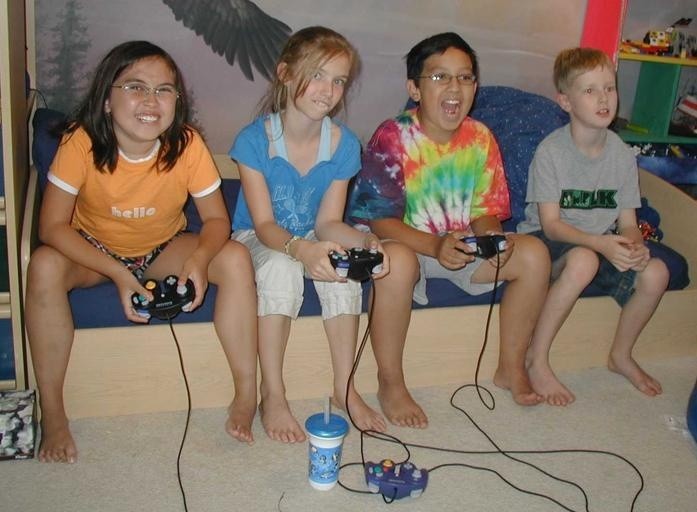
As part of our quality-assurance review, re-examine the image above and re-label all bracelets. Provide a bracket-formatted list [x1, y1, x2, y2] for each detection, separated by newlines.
[283, 234, 302, 256]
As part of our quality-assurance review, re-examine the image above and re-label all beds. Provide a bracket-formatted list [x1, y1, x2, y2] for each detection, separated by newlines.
[20, 153, 696, 420]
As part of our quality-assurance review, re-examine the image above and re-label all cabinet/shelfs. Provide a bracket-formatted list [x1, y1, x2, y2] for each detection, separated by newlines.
[617, 54, 697, 145]
[0, 1, 37, 392]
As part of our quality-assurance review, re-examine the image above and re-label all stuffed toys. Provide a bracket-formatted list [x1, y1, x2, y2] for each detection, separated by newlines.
[620, 23, 678, 57]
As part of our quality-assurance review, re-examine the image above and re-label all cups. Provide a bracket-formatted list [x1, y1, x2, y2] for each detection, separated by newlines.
[305, 414, 349, 489]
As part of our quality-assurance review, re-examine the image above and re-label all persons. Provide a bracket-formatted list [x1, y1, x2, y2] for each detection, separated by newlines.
[515, 44, 672, 408]
[225, 23, 389, 444]
[23, 38, 259, 466]
[344, 30, 553, 428]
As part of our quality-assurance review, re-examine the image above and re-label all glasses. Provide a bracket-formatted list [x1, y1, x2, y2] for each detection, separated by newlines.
[111, 82, 182, 100]
[416, 72, 475, 84]
